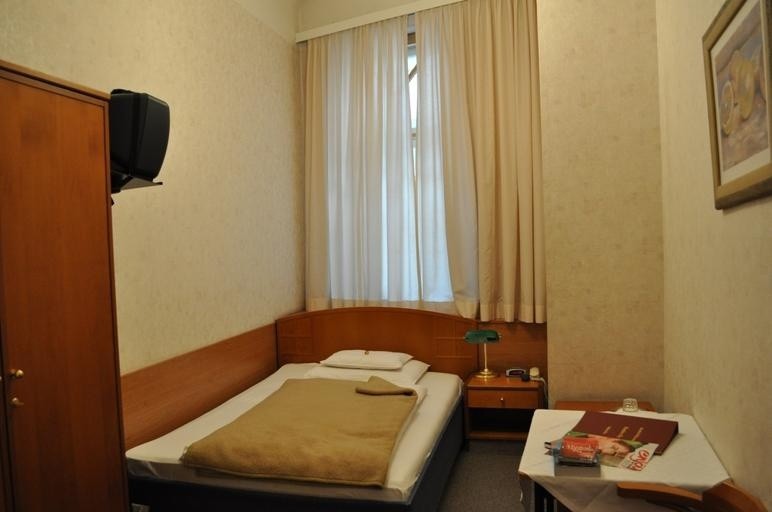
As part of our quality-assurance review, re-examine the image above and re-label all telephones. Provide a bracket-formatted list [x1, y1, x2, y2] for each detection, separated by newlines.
[528, 367, 549, 399]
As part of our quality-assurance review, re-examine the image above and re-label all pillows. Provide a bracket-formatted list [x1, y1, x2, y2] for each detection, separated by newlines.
[307, 360, 430, 387]
[320, 350, 416, 371]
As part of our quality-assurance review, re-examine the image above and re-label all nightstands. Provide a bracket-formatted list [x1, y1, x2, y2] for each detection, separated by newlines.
[462, 369, 543, 454]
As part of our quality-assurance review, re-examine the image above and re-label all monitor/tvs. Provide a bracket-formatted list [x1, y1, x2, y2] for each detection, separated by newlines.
[110, 88, 171, 181]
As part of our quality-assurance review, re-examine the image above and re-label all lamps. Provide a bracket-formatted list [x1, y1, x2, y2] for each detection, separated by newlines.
[464, 329, 500, 379]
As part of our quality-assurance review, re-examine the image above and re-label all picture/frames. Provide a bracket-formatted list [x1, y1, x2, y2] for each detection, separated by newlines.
[701, 0, 768, 208]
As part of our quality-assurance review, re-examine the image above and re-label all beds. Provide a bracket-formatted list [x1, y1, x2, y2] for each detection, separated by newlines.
[150, 306, 479, 512]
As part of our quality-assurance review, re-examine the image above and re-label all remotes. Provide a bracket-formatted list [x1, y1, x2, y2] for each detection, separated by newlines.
[521, 373, 531, 383]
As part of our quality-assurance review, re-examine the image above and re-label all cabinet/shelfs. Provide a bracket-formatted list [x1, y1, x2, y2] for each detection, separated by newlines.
[3, 61, 130, 512]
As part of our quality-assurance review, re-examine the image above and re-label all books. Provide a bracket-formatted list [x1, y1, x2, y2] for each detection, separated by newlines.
[545, 409, 679, 470]
